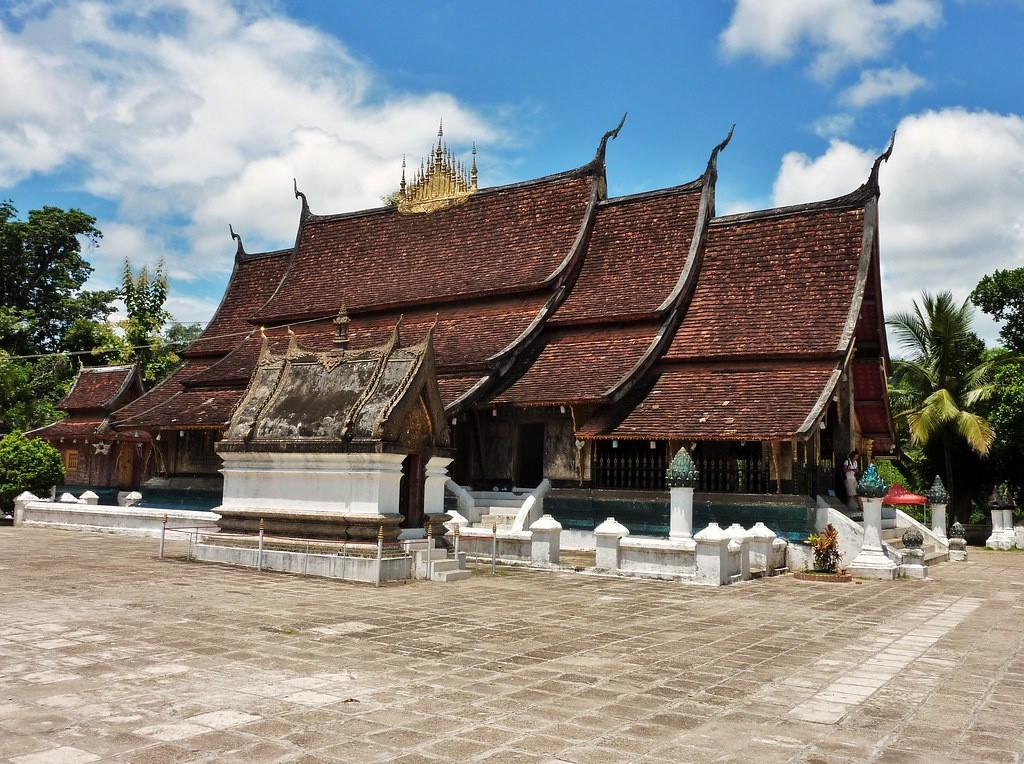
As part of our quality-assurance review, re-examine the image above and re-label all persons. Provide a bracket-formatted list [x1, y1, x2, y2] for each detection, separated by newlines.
[843, 451, 860, 496]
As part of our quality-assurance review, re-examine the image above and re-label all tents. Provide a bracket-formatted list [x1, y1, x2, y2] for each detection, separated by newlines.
[883, 482, 927, 525]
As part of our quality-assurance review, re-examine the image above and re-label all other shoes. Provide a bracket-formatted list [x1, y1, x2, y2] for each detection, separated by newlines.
[848, 509, 855, 511]
[857, 507, 863, 511]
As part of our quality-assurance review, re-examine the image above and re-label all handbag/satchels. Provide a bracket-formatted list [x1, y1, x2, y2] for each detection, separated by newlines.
[854, 469, 863, 477]
[841, 459, 850, 480]
